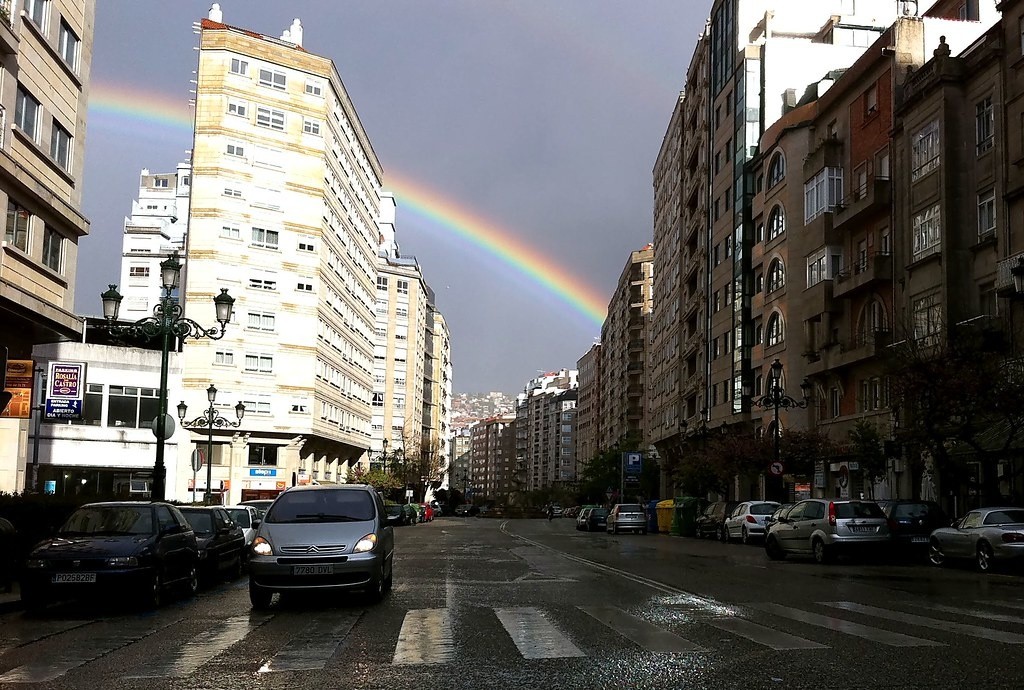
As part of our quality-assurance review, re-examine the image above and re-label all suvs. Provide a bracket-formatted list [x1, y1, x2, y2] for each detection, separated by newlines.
[606, 504, 647, 535]
[766, 498, 888, 560]
[873, 500, 948, 551]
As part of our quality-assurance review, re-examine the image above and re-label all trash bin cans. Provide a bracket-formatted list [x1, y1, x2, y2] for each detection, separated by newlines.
[644, 496, 709, 537]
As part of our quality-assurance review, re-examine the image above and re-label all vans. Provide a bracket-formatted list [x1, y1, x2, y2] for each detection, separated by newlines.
[247, 485, 393, 610]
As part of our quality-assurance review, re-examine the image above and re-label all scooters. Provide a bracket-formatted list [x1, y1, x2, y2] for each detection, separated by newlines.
[548, 512, 552, 521]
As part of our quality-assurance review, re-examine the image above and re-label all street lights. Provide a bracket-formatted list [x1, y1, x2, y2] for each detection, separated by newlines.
[102, 253, 235, 507]
[178, 386, 246, 504]
[368, 439, 402, 477]
[677, 358, 813, 500]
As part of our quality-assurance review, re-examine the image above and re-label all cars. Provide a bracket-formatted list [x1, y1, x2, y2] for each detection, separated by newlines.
[224, 505, 260, 553]
[696, 500, 781, 541]
[552, 506, 562, 517]
[385, 504, 417, 525]
[418, 505, 443, 521]
[175, 504, 245, 581]
[564, 505, 609, 530]
[237, 500, 275, 522]
[455, 505, 480, 517]
[24, 501, 197, 601]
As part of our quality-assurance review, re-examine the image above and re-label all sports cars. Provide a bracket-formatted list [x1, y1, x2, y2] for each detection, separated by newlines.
[929, 507, 1024, 569]
